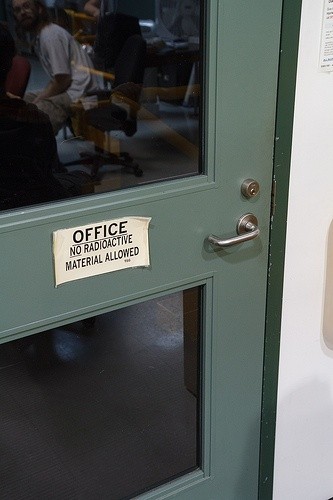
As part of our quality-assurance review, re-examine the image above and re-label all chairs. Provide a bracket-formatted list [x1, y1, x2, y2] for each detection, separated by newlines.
[5, 54, 32, 101]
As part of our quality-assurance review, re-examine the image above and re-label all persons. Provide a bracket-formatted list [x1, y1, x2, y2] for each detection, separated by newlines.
[0, 26, 94, 214]
[11, 0, 97, 175]
[83, 0, 100, 18]
